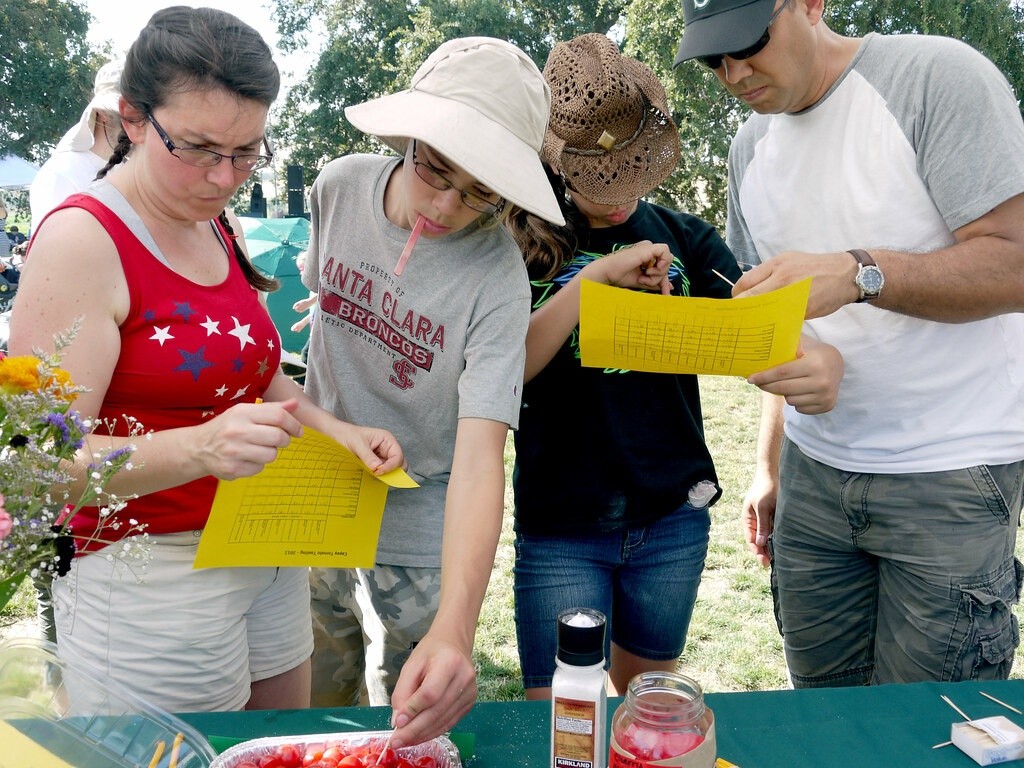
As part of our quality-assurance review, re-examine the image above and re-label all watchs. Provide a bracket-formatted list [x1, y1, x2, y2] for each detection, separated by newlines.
[847, 249, 884, 305]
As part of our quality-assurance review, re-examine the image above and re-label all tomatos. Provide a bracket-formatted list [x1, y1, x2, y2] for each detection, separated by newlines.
[233, 745, 440, 768]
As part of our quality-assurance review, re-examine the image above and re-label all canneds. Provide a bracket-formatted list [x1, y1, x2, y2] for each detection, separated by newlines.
[609, 671, 716, 768]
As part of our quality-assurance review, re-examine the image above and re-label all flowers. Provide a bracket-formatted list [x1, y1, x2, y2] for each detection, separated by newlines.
[0, 312, 153, 638]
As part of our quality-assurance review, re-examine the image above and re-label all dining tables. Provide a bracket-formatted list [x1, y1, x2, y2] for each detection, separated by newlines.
[7, 676, 1024, 768]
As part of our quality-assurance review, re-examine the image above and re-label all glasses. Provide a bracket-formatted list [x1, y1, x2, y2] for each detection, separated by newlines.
[694, 0, 790, 70]
[146, 112, 273, 172]
[413, 138, 507, 216]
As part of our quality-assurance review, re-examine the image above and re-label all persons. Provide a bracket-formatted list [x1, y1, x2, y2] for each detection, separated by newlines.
[672, 2, 1024, 691]
[1, 203, 31, 311]
[8, 5, 411, 718]
[28, 61, 136, 716]
[505, 35, 844, 703]
[296, 36, 567, 746]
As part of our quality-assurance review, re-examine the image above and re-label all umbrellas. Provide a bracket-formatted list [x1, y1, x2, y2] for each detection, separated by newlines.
[237, 216, 316, 369]
[1, 153, 43, 191]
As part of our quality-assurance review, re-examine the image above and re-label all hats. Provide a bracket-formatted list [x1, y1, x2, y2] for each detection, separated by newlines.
[344, 36, 567, 227]
[55, 59, 123, 153]
[672, 0, 777, 70]
[541, 33, 680, 206]
